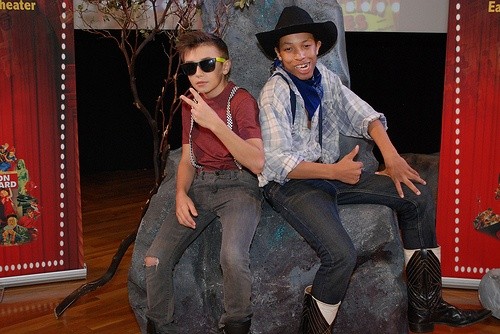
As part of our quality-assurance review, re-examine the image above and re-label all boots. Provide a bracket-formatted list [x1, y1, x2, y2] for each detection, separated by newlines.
[302, 285, 342, 334]
[405, 247, 493, 334]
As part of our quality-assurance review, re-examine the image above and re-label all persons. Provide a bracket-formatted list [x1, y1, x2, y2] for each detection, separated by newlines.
[144, 31, 266, 334]
[255, 6, 492, 334]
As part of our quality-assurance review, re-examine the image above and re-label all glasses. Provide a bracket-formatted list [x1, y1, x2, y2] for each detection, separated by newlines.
[181, 57, 226, 76]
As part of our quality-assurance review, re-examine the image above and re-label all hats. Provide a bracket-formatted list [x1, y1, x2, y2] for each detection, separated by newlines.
[255, 6, 339, 61]
